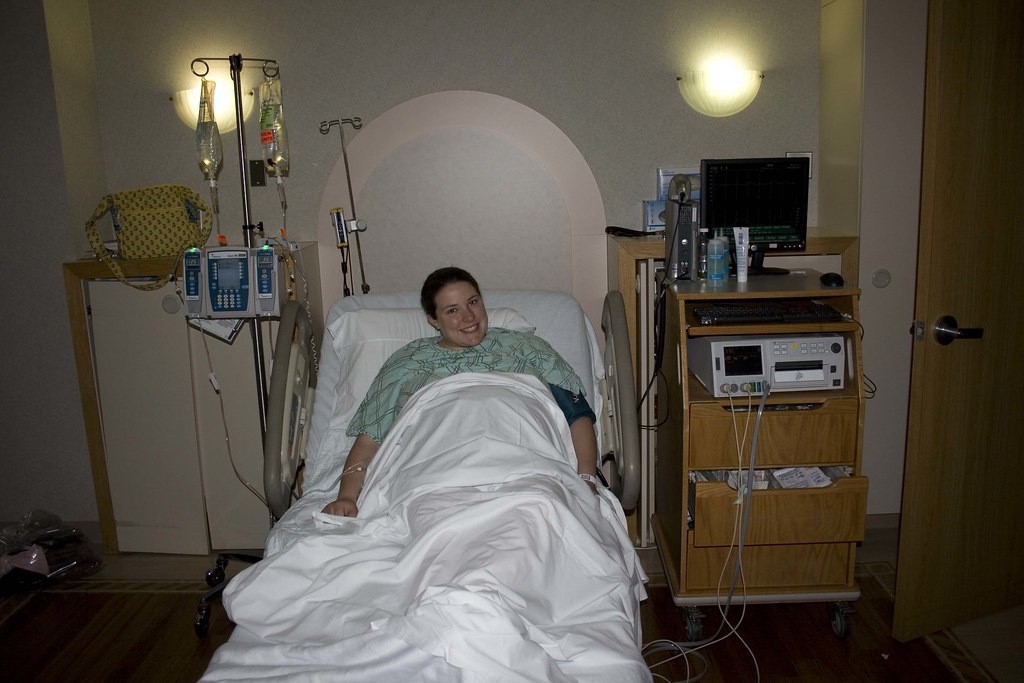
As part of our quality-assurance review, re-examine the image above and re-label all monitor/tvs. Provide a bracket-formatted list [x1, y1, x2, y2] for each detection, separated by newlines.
[701, 157, 810, 276]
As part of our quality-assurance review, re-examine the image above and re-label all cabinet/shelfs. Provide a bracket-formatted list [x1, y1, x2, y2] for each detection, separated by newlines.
[62, 241, 324, 557]
[651, 268, 868, 607]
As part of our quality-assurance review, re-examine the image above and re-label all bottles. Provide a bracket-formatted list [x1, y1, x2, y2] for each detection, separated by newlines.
[706, 228, 729, 287]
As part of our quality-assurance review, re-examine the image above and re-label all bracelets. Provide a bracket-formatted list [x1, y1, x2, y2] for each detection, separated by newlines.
[576, 474, 598, 484]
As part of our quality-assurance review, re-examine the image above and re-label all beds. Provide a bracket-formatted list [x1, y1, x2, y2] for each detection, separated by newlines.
[195, 289, 654, 683]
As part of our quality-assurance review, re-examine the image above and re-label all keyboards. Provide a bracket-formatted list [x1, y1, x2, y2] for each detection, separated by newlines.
[693, 303, 843, 324]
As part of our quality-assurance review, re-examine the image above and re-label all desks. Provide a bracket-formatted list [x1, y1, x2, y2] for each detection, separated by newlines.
[608, 226, 861, 548]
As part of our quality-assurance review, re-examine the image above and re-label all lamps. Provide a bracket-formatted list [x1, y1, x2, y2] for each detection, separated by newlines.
[676, 69, 765, 118]
[168, 86, 255, 135]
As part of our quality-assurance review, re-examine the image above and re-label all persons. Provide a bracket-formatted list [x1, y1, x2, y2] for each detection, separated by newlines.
[320, 268, 598, 520]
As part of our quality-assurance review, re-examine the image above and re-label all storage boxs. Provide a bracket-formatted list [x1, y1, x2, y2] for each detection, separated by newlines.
[642, 201, 696, 232]
[656, 167, 700, 201]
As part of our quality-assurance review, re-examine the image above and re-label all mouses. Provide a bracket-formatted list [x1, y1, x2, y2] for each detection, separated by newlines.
[820, 272, 844, 287]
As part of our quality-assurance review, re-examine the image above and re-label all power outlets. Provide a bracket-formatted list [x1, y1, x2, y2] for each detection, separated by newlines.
[784, 152, 813, 179]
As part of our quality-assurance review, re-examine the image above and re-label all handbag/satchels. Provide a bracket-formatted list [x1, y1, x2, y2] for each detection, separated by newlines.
[85, 185, 213, 291]
